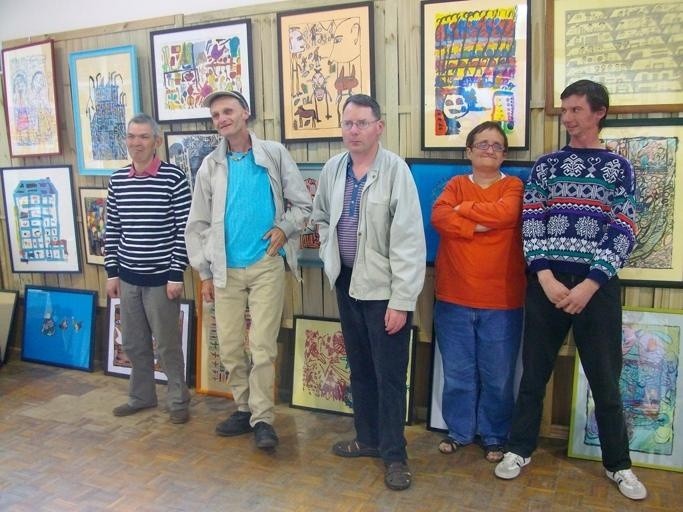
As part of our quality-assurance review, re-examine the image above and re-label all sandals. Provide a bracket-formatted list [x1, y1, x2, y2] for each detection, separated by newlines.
[484, 449, 504, 464]
[439, 438, 465, 454]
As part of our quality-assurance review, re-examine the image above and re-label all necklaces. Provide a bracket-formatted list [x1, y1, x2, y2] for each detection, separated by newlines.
[226, 147, 252, 161]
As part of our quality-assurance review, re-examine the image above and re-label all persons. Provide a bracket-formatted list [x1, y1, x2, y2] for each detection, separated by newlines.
[104, 113, 192, 426]
[494, 79, 647, 500]
[184, 89, 312, 452]
[430, 120, 525, 464]
[311, 94, 426, 491]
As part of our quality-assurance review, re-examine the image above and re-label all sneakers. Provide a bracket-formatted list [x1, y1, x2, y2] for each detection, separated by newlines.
[383, 459, 412, 491]
[113, 403, 139, 416]
[605, 467, 648, 500]
[252, 421, 279, 449]
[168, 403, 188, 424]
[215, 411, 252, 437]
[494, 452, 533, 480]
[332, 438, 381, 457]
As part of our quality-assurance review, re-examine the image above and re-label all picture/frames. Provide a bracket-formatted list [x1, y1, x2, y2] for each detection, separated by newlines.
[149, 18, 256, 123]
[568, 308, 683, 472]
[163, 129, 224, 191]
[21, 284, 98, 372]
[276, 0, 374, 144]
[1, 39, 62, 158]
[0, 289, 20, 363]
[544, 0, 683, 116]
[419, 0, 531, 152]
[195, 280, 275, 403]
[566, 118, 683, 288]
[404, 158, 535, 267]
[289, 315, 417, 426]
[68, 44, 142, 177]
[78, 186, 108, 267]
[426, 288, 450, 433]
[282, 162, 325, 268]
[104, 293, 194, 388]
[0, 164, 81, 273]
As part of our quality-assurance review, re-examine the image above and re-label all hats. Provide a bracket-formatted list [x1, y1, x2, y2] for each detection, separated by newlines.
[202, 89, 250, 113]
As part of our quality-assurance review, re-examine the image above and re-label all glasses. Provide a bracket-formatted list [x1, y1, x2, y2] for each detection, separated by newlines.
[340, 119, 380, 131]
[467, 140, 507, 152]
